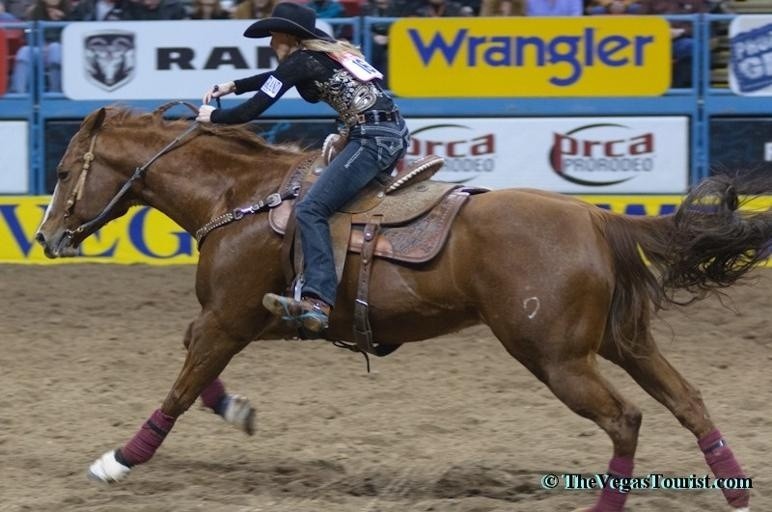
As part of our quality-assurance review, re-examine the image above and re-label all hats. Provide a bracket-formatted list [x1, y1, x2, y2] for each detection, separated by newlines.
[243, 3, 337, 44]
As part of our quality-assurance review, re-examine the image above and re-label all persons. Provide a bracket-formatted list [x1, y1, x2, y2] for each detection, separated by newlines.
[3, 2, 731, 99]
[194, 2, 412, 333]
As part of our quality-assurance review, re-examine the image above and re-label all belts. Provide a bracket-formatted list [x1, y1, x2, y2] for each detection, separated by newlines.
[357, 110, 399, 123]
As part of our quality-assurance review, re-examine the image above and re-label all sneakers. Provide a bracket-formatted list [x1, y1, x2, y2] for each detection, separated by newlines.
[263, 292, 330, 332]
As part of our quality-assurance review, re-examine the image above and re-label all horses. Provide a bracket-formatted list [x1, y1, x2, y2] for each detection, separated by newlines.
[34, 103, 772, 512]
